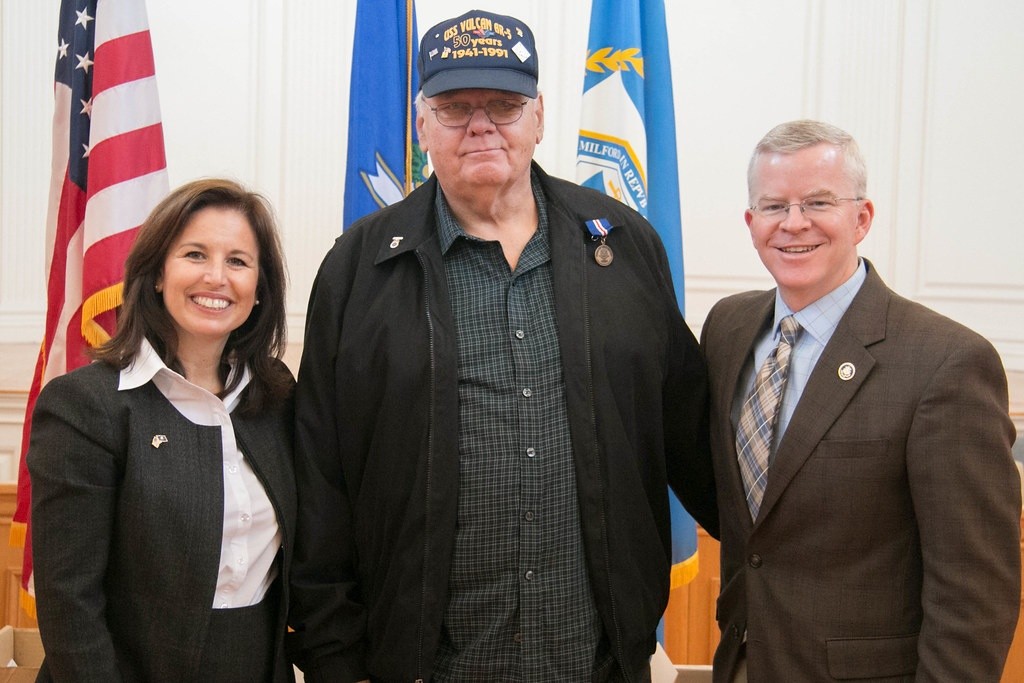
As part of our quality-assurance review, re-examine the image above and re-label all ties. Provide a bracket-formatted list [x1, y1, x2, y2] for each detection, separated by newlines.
[735, 316, 803, 525]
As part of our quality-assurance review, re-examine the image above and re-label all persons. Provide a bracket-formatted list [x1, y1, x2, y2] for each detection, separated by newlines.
[294, 10, 721, 682]
[701, 121, 1023, 683]
[25, 178, 295, 683]
[9, 1, 172, 601]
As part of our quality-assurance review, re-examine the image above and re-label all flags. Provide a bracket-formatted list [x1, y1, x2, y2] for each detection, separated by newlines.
[344, 0, 430, 235]
[570, 1, 700, 645]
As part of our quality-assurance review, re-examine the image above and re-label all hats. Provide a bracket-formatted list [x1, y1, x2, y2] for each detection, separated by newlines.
[416, 10, 540, 101]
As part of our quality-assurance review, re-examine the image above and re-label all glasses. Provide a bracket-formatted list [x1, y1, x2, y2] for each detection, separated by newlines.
[749, 194, 864, 218]
[420, 95, 531, 128]
